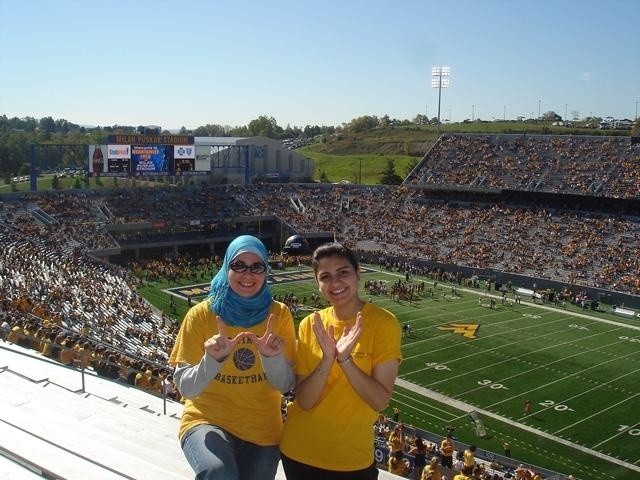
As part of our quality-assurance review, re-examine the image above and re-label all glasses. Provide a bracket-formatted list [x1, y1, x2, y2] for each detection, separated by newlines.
[229, 263, 268, 273]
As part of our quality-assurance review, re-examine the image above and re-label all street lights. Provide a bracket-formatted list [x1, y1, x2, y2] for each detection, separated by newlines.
[431, 66, 450, 138]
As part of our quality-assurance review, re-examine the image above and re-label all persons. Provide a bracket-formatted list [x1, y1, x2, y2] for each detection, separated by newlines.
[168, 235, 296, 479]
[1, 132, 638, 425]
[374, 414, 574, 480]
[279, 243, 403, 480]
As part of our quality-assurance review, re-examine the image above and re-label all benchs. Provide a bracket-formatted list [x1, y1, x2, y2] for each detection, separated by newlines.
[1, 185, 397, 257]
[0, 226, 196, 480]
[373, 419, 538, 479]
[397, 134, 639, 297]
